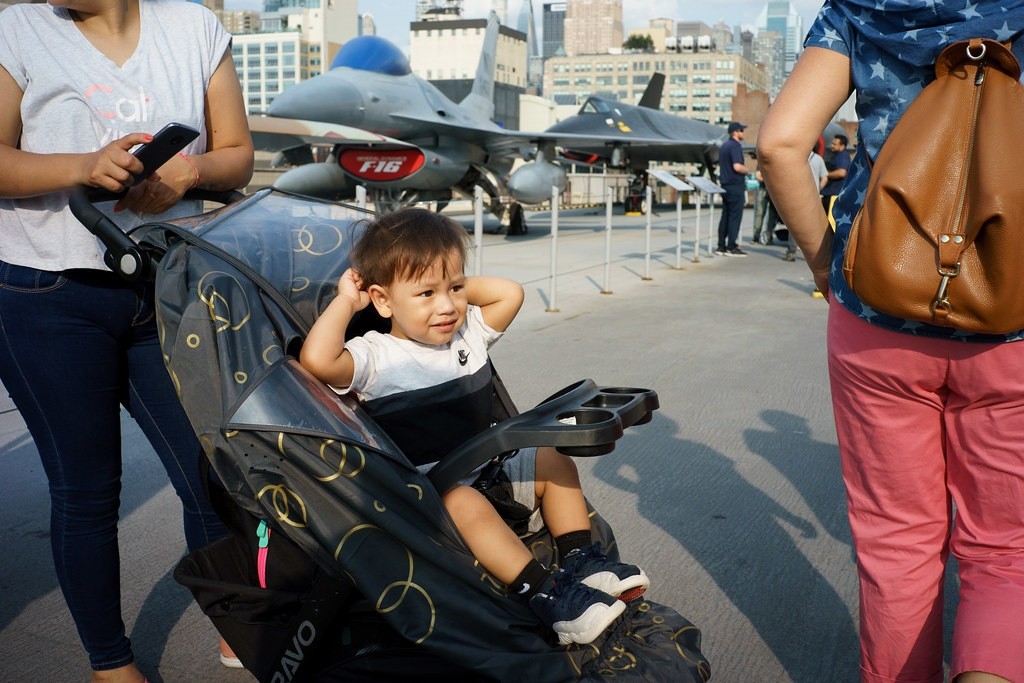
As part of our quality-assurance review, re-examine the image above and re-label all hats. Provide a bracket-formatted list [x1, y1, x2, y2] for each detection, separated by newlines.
[727, 121, 749, 133]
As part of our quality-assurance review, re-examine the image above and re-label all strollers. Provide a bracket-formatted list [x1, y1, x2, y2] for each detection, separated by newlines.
[68, 170, 711, 683]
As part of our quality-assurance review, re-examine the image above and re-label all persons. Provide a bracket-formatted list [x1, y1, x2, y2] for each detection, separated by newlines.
[299, 207, 650, 644]
[0, 1, 255, 681]
[751, 134, 850, 261]
[716, 122, 750, 257]
[757, 0, 1024, 682]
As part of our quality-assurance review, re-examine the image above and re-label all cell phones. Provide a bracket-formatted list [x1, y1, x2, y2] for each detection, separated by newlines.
[132, 123, 200, 186]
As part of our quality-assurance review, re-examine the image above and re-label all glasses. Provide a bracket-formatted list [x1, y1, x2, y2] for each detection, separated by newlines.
[735, 129, 745, 133]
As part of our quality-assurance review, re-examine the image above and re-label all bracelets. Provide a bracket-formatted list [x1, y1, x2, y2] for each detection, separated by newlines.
[178, 151, 202, 189]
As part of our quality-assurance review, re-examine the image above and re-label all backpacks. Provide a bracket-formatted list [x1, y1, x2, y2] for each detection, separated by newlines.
[846, 31, 1024, 336]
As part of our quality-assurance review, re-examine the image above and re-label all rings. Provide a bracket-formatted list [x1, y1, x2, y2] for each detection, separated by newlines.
[147, 190, 157, 200]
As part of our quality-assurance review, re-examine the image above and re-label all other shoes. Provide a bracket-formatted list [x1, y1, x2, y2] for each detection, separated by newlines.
[812, 287, 826, 299]
[216, 632, 245, 672]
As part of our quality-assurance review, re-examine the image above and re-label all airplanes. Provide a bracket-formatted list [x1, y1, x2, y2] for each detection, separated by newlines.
[262, 10, 545, 236]
[527, 70, 759, 215]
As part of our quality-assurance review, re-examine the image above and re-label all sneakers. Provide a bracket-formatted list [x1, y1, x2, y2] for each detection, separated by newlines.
[786, 252, 797, 261]
[530, 562, 626, 646]
[722, 244, 748, 258]
[715, 243, 726, 255]
[559, 540, 650, 603]
